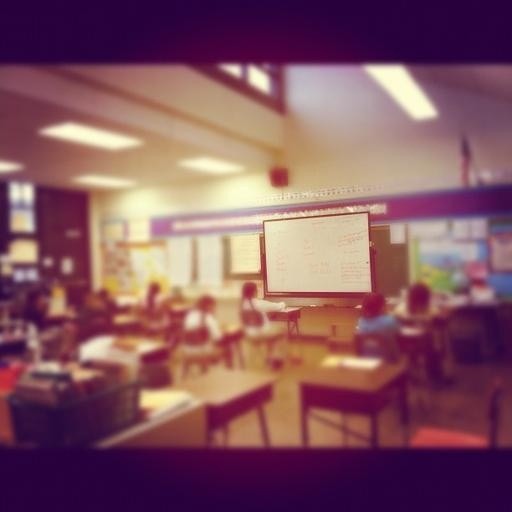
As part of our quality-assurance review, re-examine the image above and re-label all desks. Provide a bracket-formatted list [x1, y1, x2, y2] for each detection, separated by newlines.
[178, 304, 411, 449]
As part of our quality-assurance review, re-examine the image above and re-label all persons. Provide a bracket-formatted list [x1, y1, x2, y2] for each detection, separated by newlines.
[358, 290, 400, 364]
[240, 280, 287, 367]
[136, 278, 167, 330]
[396, 278, 460, 389]
[179, 292, 235, 365]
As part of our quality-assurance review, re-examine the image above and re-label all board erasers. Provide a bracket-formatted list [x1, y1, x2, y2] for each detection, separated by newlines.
[320, 304, 334, 307]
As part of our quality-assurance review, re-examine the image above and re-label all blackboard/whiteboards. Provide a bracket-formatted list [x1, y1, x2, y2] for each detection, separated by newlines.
[222, 223, 410, 307]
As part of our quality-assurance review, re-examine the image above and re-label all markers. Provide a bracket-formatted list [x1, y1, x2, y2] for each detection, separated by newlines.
[309, 305, 316, 306]
